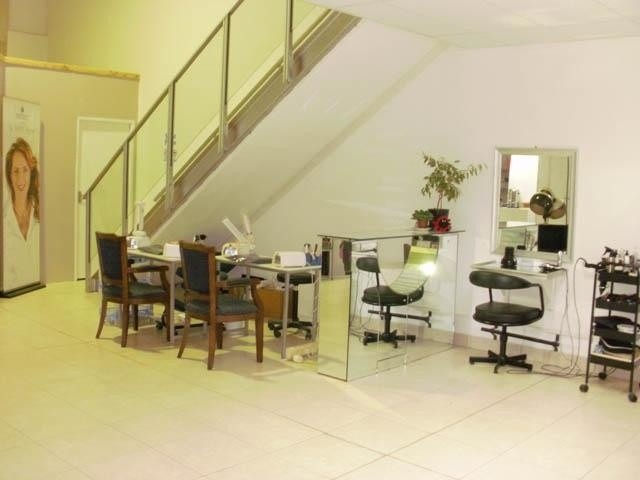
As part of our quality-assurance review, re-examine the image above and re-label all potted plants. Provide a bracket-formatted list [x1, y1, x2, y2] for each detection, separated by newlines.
[411, 210, 433, 228]
[418, 152, 487, 218]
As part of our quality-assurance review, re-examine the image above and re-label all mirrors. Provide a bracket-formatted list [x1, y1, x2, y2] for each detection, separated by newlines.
[490, 145, 576, 262]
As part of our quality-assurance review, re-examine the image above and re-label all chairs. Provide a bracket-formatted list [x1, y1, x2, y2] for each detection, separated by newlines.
[177, 241, 265, 370]
[95, 232, 170, 346]
[357, 234, 441, 347]
[469, 271, 545, 372]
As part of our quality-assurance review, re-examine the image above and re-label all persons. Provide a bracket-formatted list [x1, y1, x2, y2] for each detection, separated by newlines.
[3, 136, 40, 292]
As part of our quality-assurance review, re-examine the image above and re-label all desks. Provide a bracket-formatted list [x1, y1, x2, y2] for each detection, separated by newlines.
[318, 229, 465, 346]
[215, 254, 321, 358]
[127, 248, 222, 345]
